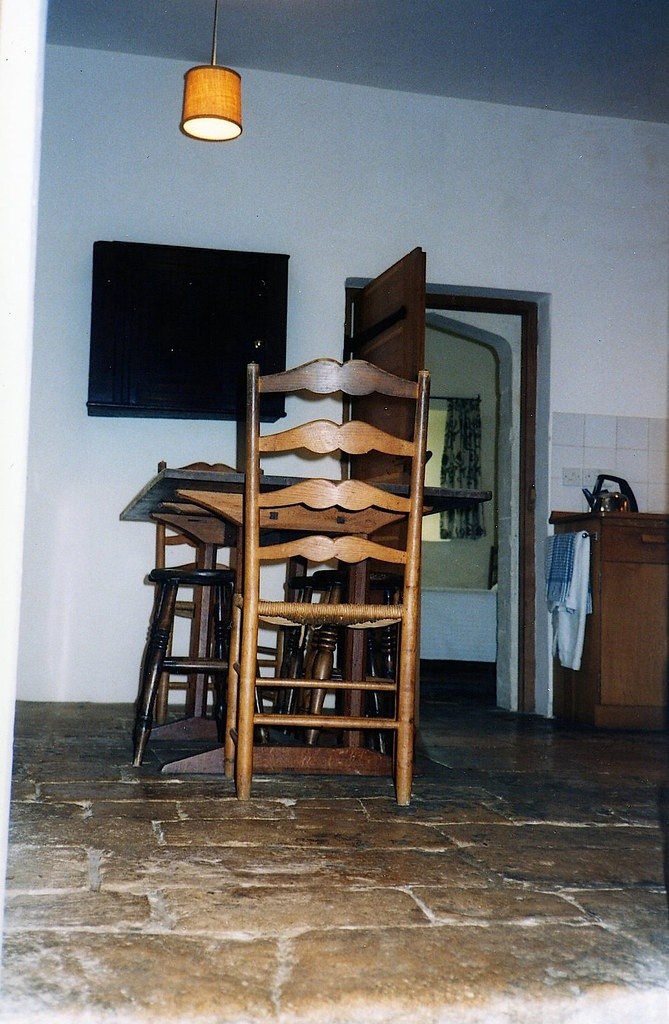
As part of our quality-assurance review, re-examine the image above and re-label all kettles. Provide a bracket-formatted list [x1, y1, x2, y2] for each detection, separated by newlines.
[582, 473, 639, 513]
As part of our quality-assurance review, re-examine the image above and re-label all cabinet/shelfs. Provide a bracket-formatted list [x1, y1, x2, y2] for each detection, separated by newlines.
[548, 511, 669, 732]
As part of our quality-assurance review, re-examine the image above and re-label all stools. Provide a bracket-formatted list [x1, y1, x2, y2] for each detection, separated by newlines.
[132, 569, 405, 768]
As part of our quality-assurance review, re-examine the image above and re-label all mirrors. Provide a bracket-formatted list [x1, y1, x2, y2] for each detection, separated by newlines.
[421, 323, 500, 589]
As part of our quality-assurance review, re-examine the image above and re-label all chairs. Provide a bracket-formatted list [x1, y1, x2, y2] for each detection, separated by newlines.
[154, 461, 285, 727]
[224, 358, 431, 807]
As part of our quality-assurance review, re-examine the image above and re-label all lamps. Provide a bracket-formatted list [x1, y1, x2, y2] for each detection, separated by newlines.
[179, 0, 242, 141]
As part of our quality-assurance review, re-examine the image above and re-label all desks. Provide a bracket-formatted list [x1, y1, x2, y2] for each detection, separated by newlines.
[120, 468, 492, 778]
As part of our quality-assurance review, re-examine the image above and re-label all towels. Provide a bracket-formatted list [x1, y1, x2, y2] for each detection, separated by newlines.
[544, 531, 589, 671]
